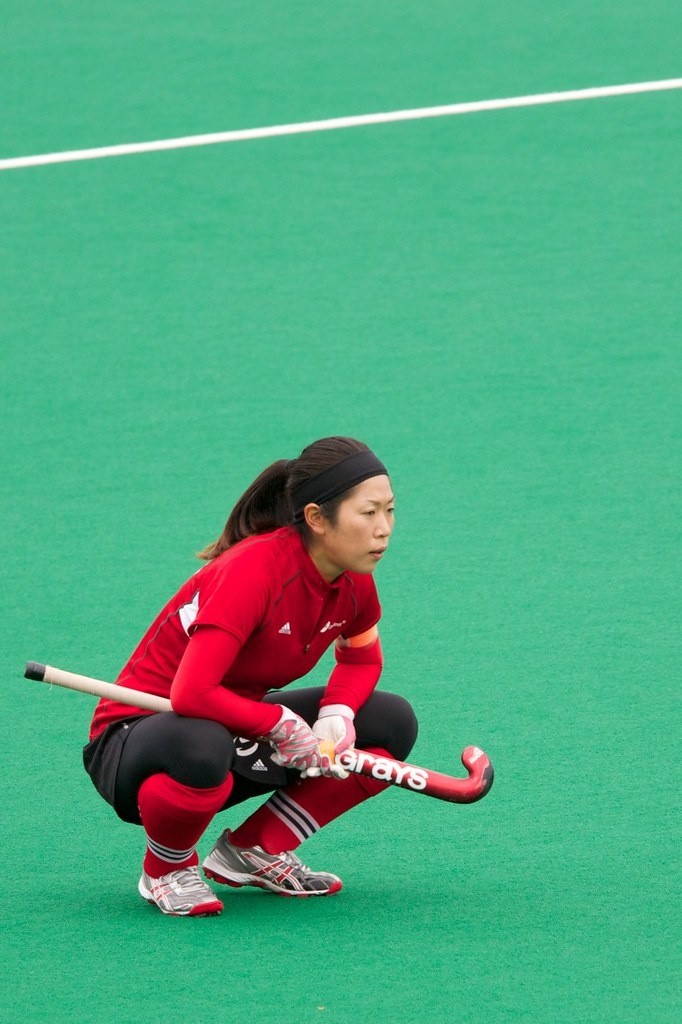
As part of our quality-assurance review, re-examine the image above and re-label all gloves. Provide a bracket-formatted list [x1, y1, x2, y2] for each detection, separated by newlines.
[260, 704, 322, 773]
[300, 715, 358, 780]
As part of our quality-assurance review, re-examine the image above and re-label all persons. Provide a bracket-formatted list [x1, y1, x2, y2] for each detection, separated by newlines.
[80, 433, 418, 918]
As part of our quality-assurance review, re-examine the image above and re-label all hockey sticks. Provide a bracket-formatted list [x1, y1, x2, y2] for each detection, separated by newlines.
[25, 660, 494, 806]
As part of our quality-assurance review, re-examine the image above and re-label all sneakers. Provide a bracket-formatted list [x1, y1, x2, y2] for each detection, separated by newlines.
[201, 828, 342, 896]
[138, 865, 224, 916]
